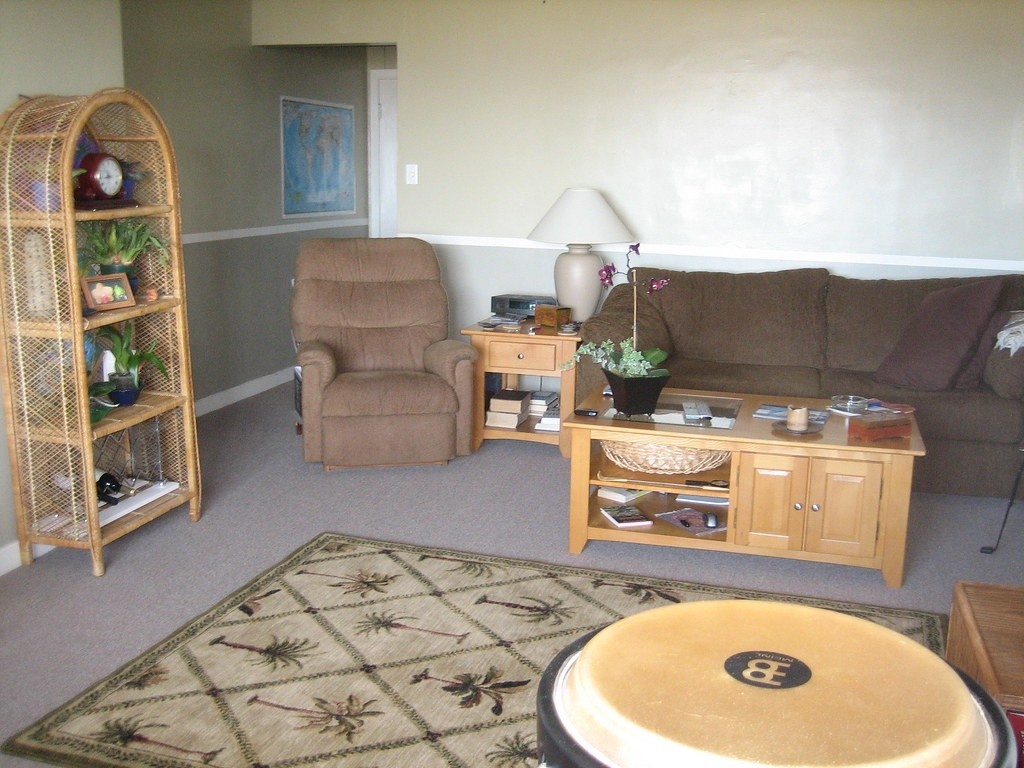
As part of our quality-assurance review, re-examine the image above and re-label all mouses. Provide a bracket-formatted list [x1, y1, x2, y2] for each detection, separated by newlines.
[703, 511, 718, 528]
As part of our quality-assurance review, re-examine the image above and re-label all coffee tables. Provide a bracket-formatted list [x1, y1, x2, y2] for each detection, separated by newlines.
[560, 388, 926, 586]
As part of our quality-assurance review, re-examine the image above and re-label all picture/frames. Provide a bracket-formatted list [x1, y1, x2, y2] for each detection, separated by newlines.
[276, 96, 359, 219]
[84, 270, 136, 311]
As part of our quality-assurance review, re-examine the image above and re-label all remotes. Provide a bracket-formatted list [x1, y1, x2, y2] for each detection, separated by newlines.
[681, 400, 713, 420]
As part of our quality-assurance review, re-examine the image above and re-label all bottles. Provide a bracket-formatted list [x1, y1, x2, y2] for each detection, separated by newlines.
[49, 453, 136, 506]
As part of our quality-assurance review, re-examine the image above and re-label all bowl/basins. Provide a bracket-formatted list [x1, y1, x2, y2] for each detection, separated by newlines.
[90, 403, 114, 424]
[831, 395, 868, 412]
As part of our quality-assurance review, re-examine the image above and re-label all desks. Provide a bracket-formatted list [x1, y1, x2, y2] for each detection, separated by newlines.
[943, 581, 1024, 716]
[533, 599, 1018, 768]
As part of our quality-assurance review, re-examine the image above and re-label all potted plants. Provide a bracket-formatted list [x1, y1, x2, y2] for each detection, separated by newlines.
[86, 319, 171, 406]
[83, 214, 162, 306]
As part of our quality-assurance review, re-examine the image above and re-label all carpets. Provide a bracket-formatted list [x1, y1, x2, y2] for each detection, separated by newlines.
[1, 534, 950, 767]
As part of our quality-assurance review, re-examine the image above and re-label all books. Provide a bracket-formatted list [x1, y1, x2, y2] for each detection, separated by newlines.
[599, 502, 654, 528]
[675, 494, 731, 506]
[753, 403, 832, 425]
[597, 485, 654, 503]
[485, 388, 561, 435]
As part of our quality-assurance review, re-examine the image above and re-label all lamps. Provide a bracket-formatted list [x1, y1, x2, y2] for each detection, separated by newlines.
[526, 186, 634, 324]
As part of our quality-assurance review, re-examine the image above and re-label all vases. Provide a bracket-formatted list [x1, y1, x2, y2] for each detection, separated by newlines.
[602, 369, 669, 420]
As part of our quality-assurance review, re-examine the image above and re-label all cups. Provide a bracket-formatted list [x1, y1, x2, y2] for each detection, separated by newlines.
[787, 404, 808, 432]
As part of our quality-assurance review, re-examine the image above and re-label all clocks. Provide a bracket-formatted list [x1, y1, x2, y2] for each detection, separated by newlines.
[83, 147, 123, 197]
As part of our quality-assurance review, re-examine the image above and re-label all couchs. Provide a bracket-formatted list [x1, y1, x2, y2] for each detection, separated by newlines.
[578, 264, 1024, 507]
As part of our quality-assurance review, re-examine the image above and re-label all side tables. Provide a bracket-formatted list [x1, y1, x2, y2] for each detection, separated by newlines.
[459, 313, 581, 458]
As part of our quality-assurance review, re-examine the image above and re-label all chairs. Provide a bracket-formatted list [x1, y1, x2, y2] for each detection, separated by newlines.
[289, 231, 483, 466]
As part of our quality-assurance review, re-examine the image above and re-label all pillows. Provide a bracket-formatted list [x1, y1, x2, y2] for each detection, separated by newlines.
[875, 276, 1004, 390]
[956, 311, 1011, 395]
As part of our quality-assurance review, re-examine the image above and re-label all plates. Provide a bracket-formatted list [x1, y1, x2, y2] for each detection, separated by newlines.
[772, 421, 824, 436]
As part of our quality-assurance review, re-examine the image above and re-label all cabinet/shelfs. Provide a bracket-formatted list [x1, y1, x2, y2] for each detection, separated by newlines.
[0, 89, 202, 575]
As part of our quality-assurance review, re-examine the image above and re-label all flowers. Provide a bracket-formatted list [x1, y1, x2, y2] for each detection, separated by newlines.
[560, 242, 673, 378]
